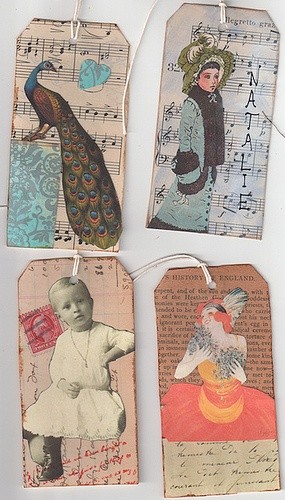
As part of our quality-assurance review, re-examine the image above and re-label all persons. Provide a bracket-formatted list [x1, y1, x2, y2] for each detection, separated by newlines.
[22, 278, 135, 480]
